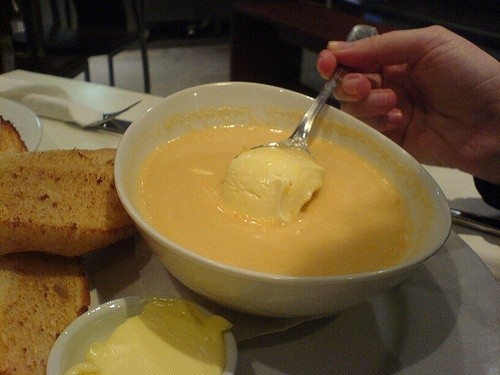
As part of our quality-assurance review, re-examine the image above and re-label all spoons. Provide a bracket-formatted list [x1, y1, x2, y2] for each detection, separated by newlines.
[237, 24, 378, 158]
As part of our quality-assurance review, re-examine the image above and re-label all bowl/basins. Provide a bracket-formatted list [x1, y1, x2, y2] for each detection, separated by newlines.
[113, 81, 452, 316]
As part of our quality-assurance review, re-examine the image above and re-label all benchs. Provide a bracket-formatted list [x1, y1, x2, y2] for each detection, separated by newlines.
[230, 0, 400, 96]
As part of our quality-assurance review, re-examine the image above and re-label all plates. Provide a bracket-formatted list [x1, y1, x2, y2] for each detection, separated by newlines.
[0, 97, 44, 153]
[45, 295, 237, 375]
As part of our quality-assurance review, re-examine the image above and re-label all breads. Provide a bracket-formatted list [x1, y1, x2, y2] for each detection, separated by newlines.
[0, 148, 138, 256]
[0, 116, 90, 375]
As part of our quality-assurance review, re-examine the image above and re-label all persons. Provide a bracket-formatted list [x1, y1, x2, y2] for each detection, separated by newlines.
[319, 25, 500, 184]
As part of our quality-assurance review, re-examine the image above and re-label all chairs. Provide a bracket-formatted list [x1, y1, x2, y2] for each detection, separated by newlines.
[0, 0, 152, 96]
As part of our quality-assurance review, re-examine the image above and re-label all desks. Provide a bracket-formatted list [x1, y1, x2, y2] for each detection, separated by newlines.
[0, 69, 498, 375]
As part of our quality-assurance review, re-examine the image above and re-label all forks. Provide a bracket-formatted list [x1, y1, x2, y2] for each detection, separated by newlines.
[87, 100, 142, 128]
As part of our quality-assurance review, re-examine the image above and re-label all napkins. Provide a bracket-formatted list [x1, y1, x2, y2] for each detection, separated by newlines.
[1, 84, 106, 127]
